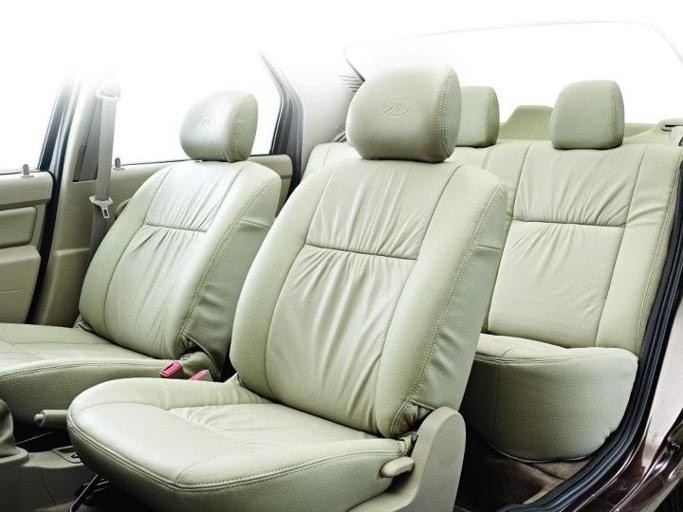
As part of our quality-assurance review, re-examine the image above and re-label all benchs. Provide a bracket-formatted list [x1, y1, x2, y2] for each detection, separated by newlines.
[299, 82, 680, 461]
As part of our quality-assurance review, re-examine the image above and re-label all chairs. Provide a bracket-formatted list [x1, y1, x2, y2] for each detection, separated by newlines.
[71, 66, 514, 510]
[0, 92, 283, 429]
